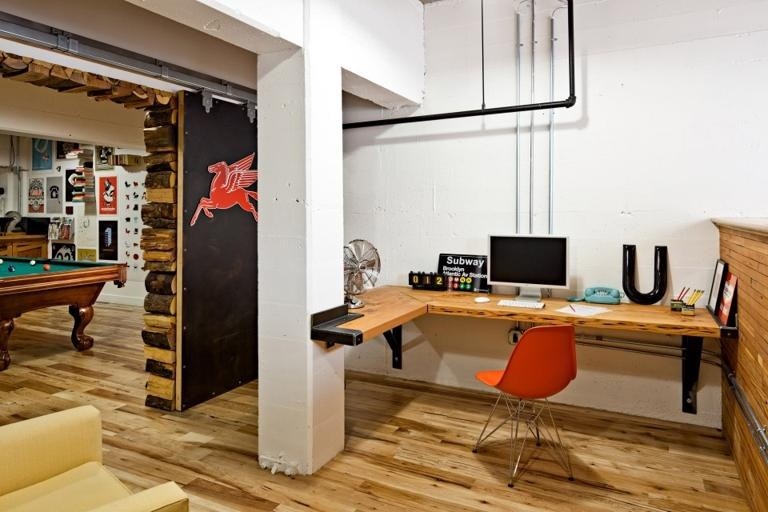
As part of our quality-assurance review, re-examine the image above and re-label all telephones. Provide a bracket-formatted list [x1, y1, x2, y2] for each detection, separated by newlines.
[584, 287, 620, 305]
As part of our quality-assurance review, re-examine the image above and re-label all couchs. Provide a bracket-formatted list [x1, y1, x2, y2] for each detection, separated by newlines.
[0, 405, 189, 511]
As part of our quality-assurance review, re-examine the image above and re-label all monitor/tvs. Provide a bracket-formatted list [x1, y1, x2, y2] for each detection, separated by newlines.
[487, 234, 569, 302]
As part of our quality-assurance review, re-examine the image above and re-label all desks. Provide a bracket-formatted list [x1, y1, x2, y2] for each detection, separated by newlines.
[0, 256, 127, 371]
[311, 285, 737, 416]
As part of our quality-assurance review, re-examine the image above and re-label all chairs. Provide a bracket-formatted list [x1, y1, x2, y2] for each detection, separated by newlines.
[471, 325, 577, 488]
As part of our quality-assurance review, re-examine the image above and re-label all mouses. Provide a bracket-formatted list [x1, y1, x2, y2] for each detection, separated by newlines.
[475, 297, 490, 303]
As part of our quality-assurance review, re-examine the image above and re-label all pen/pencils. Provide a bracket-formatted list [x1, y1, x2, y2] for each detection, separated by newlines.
[677, 287, 704, 306]
[569, 304, 575, 312]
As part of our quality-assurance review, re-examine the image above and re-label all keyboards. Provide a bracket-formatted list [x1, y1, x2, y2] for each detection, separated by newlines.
[496, 300, 545, 309]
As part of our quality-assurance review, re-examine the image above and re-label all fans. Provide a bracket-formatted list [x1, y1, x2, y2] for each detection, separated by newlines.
[344, 240, 381, 308]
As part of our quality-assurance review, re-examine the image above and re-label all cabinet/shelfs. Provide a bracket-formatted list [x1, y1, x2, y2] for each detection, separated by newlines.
[0, 231, 48, 259]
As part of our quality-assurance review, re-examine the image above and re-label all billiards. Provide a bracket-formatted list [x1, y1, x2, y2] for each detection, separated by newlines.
[30, 260, 35, 265]
[44, 264, 50, 270]
[8, 266, 15, 271]
[0, 259, 3, 264]
